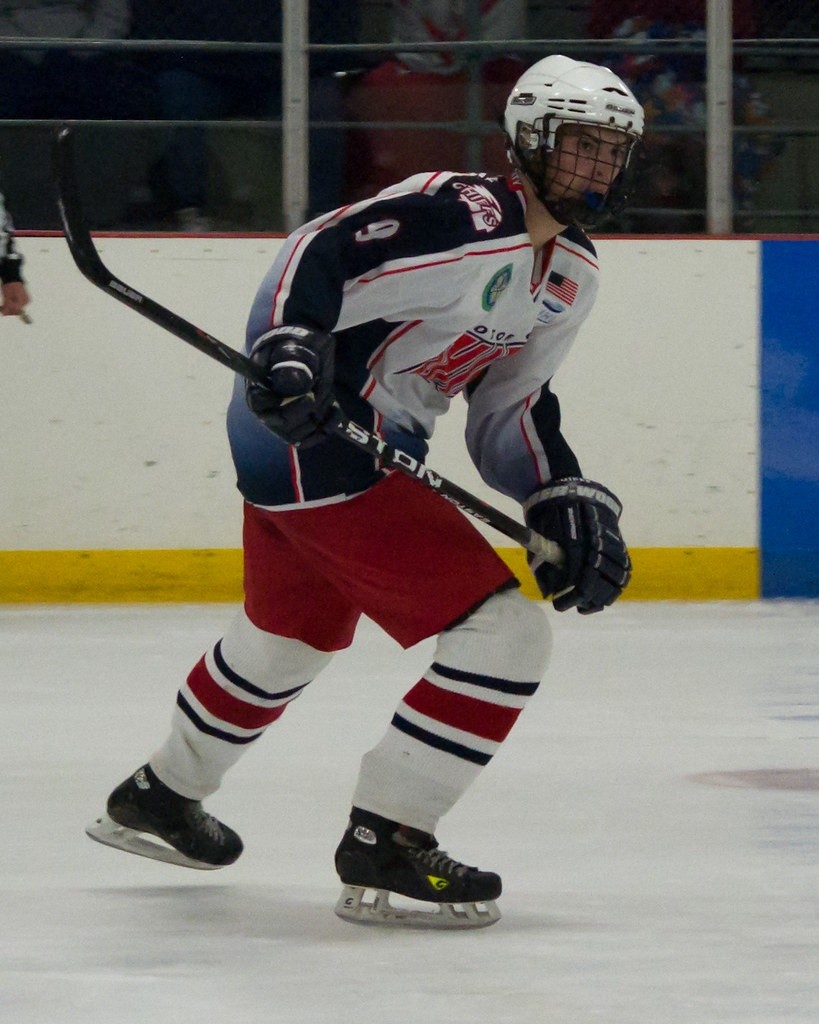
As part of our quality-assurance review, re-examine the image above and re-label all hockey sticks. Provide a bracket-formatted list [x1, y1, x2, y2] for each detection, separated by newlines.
[48, 125, 570, 572]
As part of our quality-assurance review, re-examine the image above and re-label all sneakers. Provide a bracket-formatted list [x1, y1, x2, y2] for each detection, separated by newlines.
[334, 806, 505, 931]
[86, 765, 243, 869]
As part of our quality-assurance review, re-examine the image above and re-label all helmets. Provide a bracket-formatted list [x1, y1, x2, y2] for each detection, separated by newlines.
[504, 54, 645, 236]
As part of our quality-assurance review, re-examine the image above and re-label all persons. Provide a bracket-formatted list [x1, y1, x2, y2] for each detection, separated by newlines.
[0, 192, 32, 319]
[82, 53, 643, 930]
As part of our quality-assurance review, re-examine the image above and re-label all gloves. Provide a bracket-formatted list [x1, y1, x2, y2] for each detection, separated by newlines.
[244, 324, 343, 451]
[521, 474, 631, 612]
